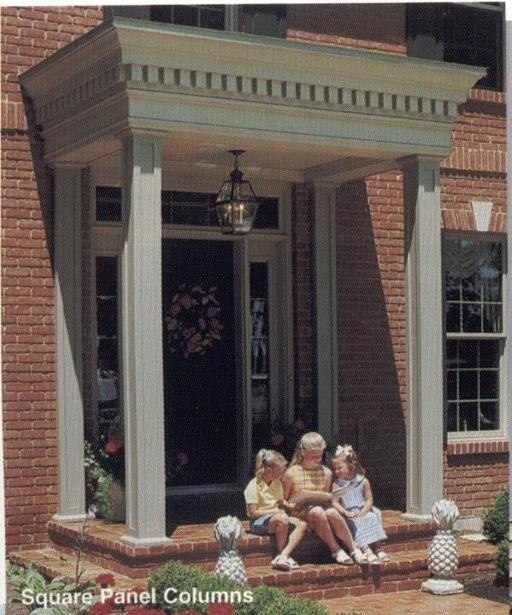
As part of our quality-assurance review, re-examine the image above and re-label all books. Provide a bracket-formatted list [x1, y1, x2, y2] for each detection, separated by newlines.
[290, 481, 353, 506]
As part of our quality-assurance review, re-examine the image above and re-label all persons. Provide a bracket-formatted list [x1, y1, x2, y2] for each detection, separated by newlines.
[242, 446, 311, 574]
[280, 430, 366, 568]
[328, 443, 394, 568]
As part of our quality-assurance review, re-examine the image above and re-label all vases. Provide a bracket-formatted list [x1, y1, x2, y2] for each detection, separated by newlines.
[95, 476, 125, 522]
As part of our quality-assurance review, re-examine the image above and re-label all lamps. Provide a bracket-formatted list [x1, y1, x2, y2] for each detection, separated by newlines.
[214, 148, 263, 237]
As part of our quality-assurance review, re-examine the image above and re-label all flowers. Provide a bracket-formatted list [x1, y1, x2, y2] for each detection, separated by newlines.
[94, 411, 188, 485]
[165, 283, 224, 358]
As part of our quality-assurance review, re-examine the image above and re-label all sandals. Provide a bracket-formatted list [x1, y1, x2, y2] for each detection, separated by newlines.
[330, 547, 354, 566]
[361, 545, 391, 564]
[349, 548, 369, 565]
[270, 556, 301, 569]
